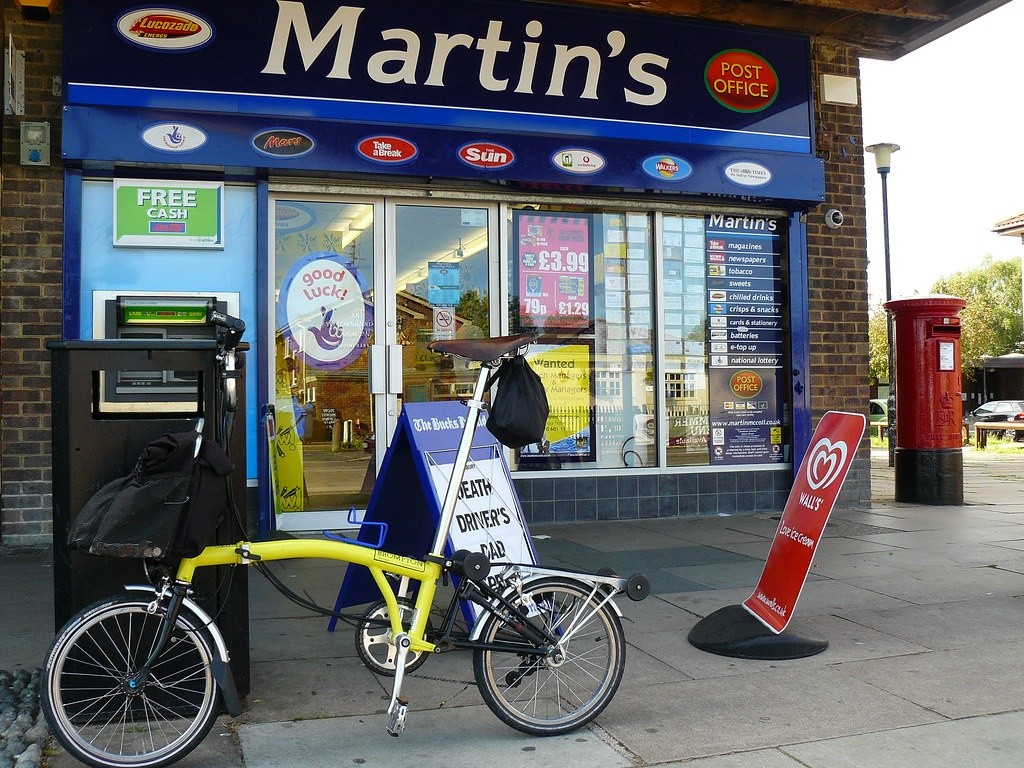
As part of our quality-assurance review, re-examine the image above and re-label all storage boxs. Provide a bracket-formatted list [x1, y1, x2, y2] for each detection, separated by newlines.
[279, 400, 316, 444]
[605, 214, 706, 390]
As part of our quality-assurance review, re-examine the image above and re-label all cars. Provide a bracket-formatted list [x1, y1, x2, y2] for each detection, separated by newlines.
[362, 431, 376, 455]
[868, 397, 890, 439]
[961, 398, 1024, 441]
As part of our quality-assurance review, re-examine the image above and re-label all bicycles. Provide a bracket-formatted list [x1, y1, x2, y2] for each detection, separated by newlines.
[36, 307, 652, 768]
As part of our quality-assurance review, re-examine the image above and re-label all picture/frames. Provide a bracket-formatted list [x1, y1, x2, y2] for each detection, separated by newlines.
[516, 338, 595, 463]
[511, 208, 595, 334]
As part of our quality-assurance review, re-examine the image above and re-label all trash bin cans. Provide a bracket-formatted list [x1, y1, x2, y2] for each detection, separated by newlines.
[46, 338, 251, 726]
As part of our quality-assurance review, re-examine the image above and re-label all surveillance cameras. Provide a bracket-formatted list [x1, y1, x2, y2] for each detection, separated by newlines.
[825, 209, 843, 229]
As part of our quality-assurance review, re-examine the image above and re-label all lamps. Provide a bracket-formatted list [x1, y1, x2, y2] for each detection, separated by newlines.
[456, 236, 462, 258]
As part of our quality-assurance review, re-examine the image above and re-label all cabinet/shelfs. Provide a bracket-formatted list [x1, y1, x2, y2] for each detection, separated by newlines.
[431, 381, 476, 400]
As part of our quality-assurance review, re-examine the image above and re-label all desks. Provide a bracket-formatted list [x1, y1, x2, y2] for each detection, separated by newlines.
[974, 422, 1024, 450]
[870, 422, 888, 441]
[962, 420, 970, 443]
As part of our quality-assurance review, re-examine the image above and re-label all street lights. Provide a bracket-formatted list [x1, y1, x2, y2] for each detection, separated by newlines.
[864, 140, 899, 468]
[978, 354, 993, 404]
